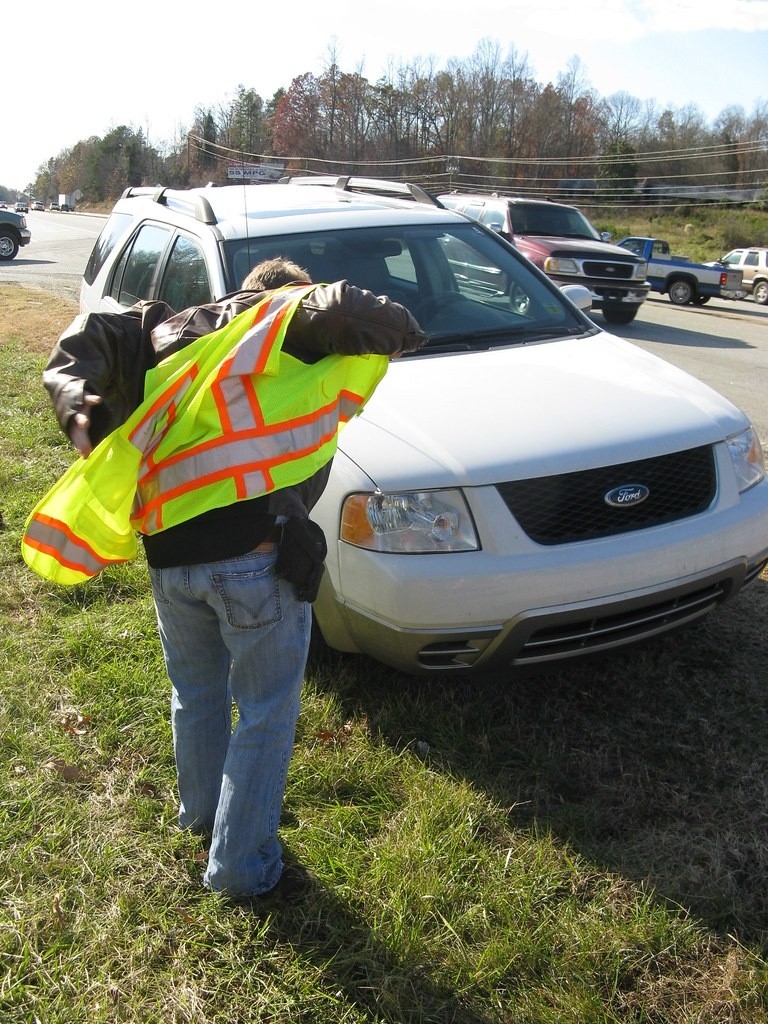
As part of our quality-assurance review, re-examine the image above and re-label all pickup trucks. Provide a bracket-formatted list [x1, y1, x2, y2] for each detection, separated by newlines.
[613, 236, 744, 305]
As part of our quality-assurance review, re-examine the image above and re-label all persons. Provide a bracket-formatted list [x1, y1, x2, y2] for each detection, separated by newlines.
[42, 256, 431, 898]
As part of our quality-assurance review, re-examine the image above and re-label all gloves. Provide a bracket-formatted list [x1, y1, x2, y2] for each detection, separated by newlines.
[273, 520, 329, 603]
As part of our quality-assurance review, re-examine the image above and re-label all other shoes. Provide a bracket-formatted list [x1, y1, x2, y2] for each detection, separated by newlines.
[261, 867, 296, 901]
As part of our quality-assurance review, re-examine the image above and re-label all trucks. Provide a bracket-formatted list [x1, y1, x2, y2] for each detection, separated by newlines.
[59, 193, 76, 212]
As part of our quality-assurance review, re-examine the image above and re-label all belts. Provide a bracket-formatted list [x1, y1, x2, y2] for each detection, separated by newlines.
[254, 543, 276, 553]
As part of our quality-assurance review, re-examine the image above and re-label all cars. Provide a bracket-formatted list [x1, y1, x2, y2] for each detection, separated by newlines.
[14, 202, 28, 213]
[697, 246, 768, 306]
[0, 201, 7, 209]
[31, 201, 45, 211]
[50, 202, 60, 211]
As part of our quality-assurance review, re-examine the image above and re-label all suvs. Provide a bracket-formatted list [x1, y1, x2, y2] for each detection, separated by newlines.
[434, 190, 652, 326]
[76, 173, 767, 681]
[0, 210, 32, 264]
